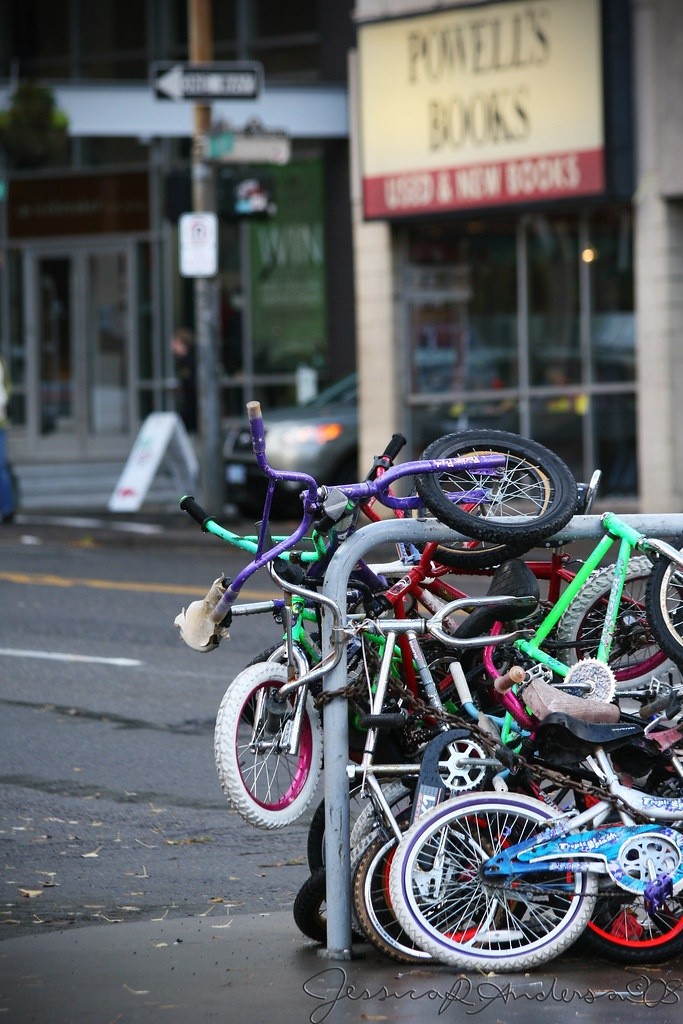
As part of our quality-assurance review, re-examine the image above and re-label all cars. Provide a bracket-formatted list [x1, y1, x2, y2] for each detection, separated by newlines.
[218, 347, 642, 519]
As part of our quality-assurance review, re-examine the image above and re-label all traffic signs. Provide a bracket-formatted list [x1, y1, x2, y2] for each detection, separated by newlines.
[149, 60, 265, 107]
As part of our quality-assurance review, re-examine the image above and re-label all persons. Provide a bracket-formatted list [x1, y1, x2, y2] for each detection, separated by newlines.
[0, 354, 19, 524]
[170, 332, 200, 436]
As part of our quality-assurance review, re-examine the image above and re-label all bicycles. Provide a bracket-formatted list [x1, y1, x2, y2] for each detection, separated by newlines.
[172, 397, 683, 977]
